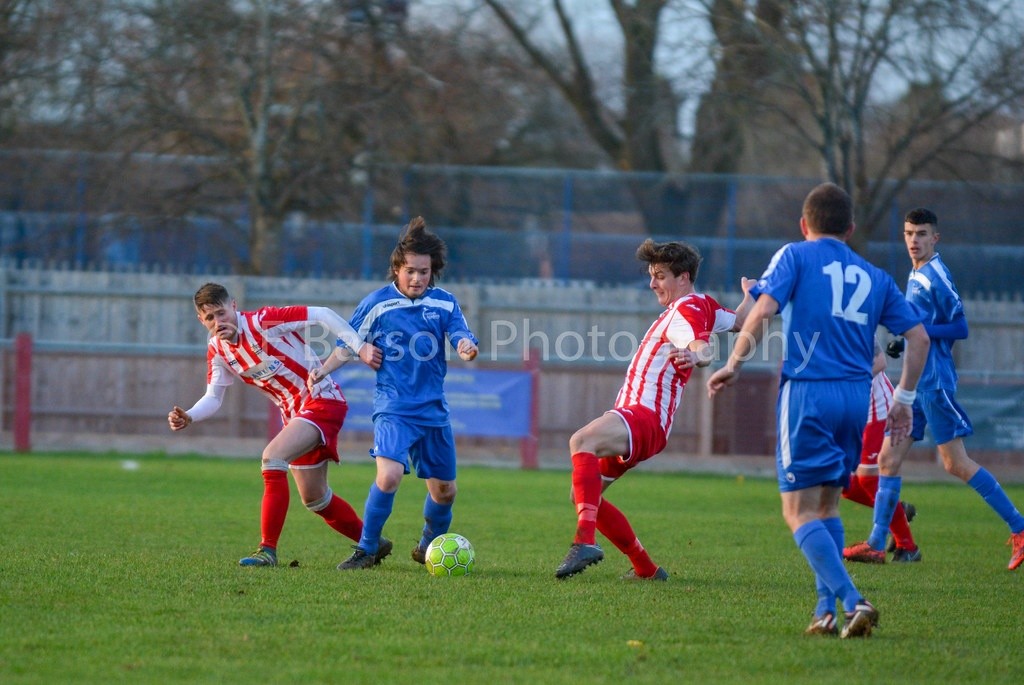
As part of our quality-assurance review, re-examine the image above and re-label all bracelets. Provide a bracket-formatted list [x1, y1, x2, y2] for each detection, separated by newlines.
[892, 384, 916, 405]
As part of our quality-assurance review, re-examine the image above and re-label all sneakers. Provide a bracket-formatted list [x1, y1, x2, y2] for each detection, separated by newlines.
[841, 541, 886, 564]
[337, 544, 376, 570]
[411, 547, 426, 564]
[891, 546, 922, 562]
[239, 547, 278, 568]
[372, 538, 393, 564]
[887, 501, 916, 552]
[807, 611, 839, 636]
[840, 598, 878, 638]
[1006, 529, 1024, 570]
[555, 543, 604, 579]
[621, 565, 668, 582]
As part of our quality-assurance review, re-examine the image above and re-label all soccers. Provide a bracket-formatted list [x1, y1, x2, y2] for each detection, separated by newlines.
[422, 534, 476, 578]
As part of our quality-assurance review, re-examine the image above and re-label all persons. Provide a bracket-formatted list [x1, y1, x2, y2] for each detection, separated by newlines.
[704, 180, 929, 637]
[838, 208, 1024, 570]
[553, 238, 759, 587]
[168, 281, 393, 565]
[839, 337, 922, 564]
[307, 216, 479, 572]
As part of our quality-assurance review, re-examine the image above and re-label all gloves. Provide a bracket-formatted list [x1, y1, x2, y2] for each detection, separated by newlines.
[886, 337, 905, 358]
